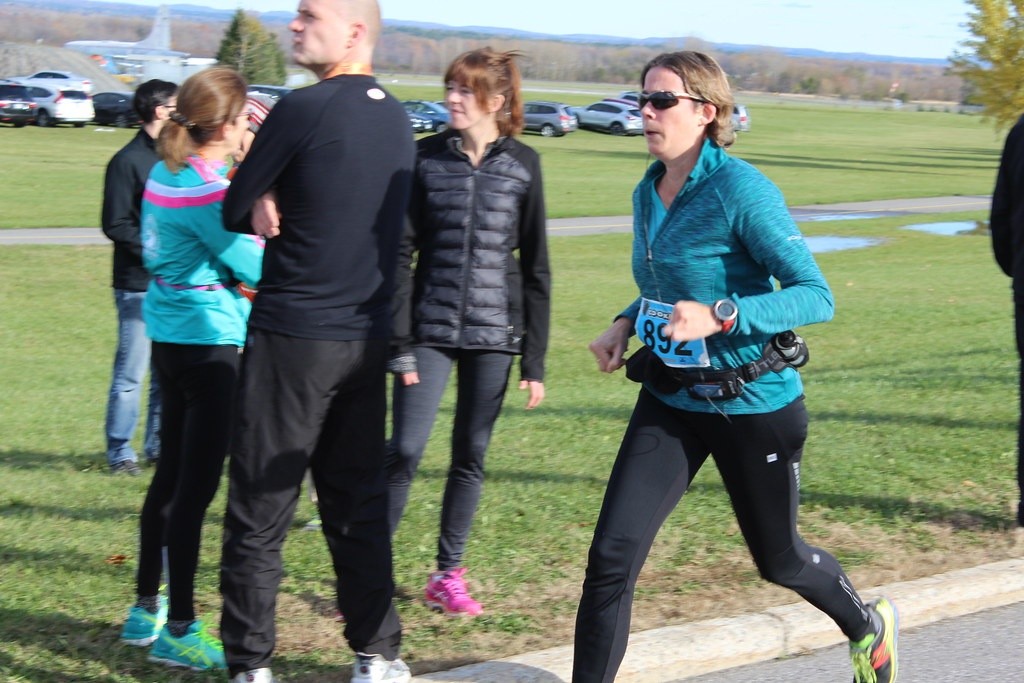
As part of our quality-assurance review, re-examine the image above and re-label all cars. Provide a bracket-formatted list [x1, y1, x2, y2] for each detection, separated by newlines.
[401, 101, 450, 135]
[247, 84, 291, 98]
[9, 77, 96, 127]
[91, 91, 144, 129]
[21, 71, 94, 93]
[602, 90, 641, 107]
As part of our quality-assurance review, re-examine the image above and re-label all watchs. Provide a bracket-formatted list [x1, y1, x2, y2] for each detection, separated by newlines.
[713, 299, 739, 333]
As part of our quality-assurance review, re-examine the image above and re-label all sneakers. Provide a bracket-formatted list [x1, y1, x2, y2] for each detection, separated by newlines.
[148, 613, 229, 671]
[350, 650, 412, 683]
[849, 594, 900, 683]
[109, 457, 141, 477]
[122, 583, 171, 647]
[306, 502, 324, 532]
[424, 567, 484, 617]
[233, 665, 273, 683]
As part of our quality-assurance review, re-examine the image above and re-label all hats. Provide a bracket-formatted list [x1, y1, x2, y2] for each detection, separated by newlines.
[240, 93, 278, 135]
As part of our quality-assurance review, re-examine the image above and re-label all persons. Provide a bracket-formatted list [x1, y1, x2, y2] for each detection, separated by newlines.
[989, 114, 1024, 531]
[572, 52, 900, 683]
[220, 0, 418, 683]
[101, 67, 276, 671]
[387, 47, 552, 617]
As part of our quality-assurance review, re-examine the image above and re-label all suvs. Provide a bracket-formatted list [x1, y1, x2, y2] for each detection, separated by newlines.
[523, 100, 579, 137]
[0, 80, 37, 129]
[732, 103, 750, 132]
[572, 101, 644, 136]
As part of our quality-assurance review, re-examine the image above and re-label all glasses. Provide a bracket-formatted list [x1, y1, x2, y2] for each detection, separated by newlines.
[637, 90, 710, 113]
[210, 106, 253, 125]
